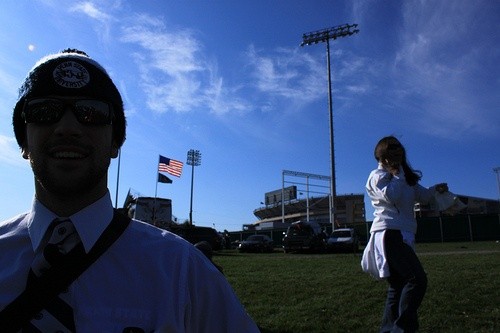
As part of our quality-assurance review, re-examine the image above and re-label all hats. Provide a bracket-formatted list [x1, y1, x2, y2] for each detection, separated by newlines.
[10, 47, 127, 154]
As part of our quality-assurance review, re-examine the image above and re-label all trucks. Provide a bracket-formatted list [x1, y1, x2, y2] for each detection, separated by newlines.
[282, 221, 323, 252]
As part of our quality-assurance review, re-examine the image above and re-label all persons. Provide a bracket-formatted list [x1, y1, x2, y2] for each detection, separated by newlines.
[0, 47, 262, 333]
[361, 136, 456, 333]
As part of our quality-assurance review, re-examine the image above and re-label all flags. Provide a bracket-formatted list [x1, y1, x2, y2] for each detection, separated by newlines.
[158, 155, 184, 178]
[158, 173, 173, 183]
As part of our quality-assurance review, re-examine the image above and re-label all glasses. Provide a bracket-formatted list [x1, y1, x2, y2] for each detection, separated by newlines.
[20, 97, 115, 130]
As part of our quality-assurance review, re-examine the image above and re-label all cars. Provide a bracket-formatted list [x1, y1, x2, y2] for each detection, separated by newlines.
[326, 228, 358, 251]
[238, 235, 274, 254]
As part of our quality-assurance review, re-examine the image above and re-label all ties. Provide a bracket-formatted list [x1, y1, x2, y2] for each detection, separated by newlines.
[29, 218, 81, 333]
